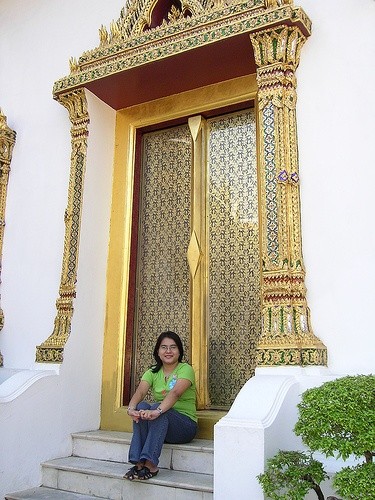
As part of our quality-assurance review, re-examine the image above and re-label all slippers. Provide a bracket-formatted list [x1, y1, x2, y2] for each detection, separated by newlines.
[123, 466, 159, 481]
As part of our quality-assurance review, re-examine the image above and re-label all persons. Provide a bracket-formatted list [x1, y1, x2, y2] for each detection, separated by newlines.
[123, 331, 198, 480]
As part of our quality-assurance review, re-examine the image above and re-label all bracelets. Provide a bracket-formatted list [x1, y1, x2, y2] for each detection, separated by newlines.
[157, 407, 162, 414]
[126, 408, 136, 415]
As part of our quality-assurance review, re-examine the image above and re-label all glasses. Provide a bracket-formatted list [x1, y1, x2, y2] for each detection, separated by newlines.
[160, 346, 177, 351]
